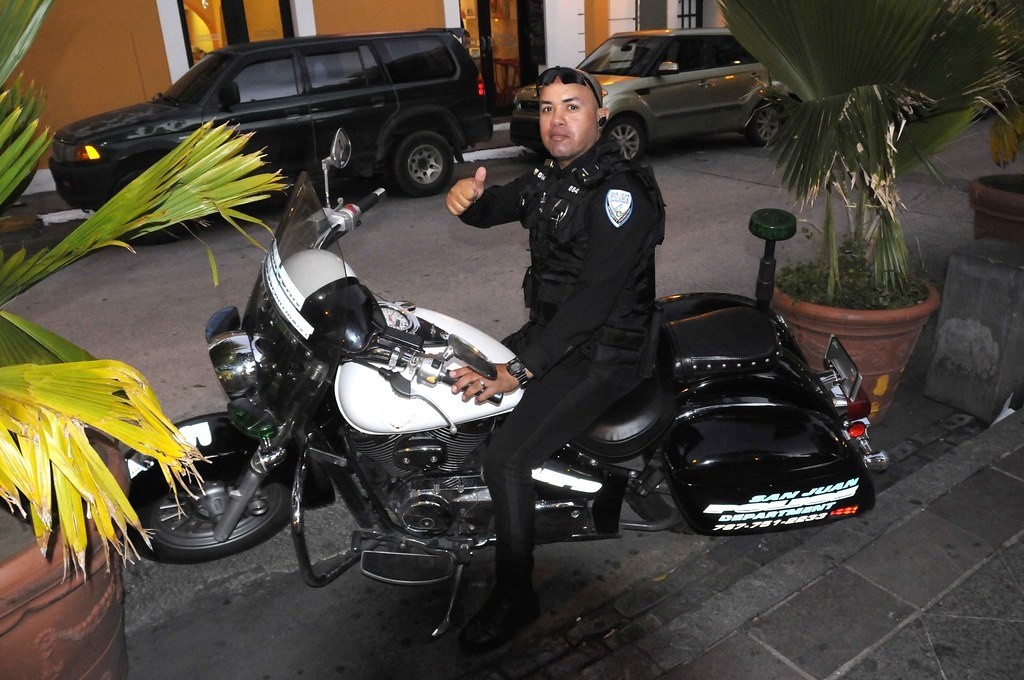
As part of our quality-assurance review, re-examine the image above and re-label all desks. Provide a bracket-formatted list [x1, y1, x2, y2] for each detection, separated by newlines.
[494, 58, 521, 107]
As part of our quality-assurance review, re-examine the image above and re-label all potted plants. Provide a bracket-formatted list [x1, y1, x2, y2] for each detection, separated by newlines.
[714, 0, 1024, 432]
[0, 1, 293, 680]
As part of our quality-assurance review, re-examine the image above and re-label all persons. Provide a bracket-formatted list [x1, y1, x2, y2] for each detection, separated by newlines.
[444, 66, 667, 657]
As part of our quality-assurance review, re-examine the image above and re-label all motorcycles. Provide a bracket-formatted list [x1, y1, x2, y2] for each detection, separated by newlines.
[122, 124, 890, 641]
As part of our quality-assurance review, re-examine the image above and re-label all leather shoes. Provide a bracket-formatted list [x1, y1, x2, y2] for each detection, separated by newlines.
[455, 588, 539, 655]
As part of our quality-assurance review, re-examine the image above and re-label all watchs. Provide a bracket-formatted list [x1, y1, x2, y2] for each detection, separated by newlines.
[506, 357, 529, 389]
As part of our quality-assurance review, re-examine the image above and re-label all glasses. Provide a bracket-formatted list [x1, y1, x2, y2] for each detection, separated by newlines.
[536, 66, 601, 110]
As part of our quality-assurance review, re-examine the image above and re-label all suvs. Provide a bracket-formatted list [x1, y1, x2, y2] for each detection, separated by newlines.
[510, 26, 792, 161]
[48, 24, 494, 243]
